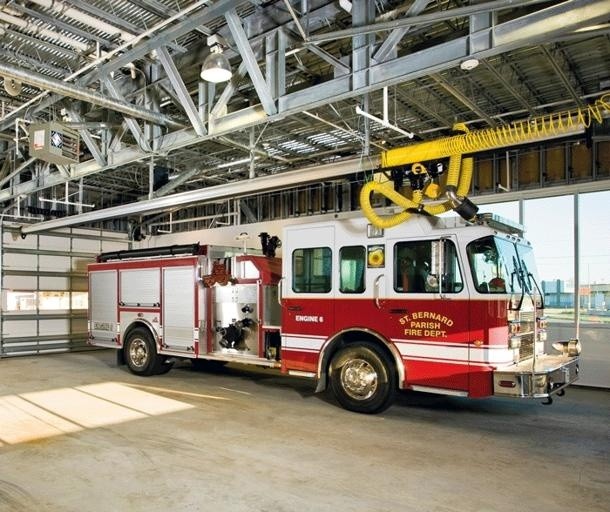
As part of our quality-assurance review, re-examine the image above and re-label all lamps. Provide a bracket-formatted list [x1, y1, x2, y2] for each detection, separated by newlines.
[200, 33, 232, 83]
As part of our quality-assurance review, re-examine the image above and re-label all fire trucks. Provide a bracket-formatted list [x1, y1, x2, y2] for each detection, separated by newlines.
[82, 203, 584, 413]
